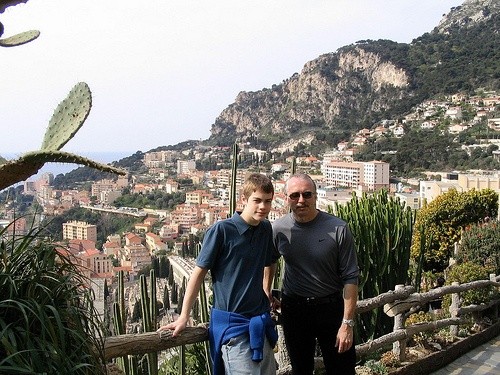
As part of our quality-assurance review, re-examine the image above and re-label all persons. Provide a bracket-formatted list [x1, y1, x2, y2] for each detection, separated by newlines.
[155, 173, 282, 375]
[263, 174, 362, 375]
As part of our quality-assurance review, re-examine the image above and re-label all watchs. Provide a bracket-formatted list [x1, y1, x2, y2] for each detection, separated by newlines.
[343, 319, 357, 327]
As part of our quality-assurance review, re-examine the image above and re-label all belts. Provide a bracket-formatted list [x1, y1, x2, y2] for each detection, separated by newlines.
[281, 289, 342, 307]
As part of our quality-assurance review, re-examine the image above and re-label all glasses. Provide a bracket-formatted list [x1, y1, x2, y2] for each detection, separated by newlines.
[288, 191, 318, 200]
